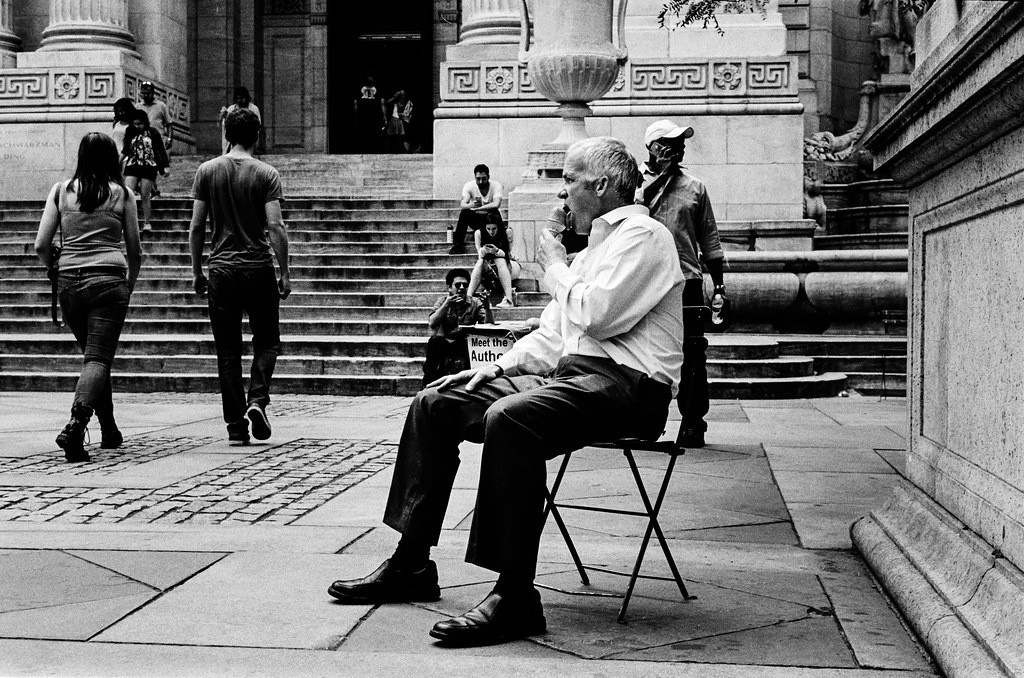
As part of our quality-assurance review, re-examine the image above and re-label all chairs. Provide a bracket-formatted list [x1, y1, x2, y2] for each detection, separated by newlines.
[533, 306, 708, 624]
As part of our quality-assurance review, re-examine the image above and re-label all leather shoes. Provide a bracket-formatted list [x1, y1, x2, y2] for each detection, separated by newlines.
[429, 589, 547, 642]
[327, 558, 441, 604]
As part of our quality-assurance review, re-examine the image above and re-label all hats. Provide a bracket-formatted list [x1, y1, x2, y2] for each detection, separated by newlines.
[141, 81, 154, 93]
[645, 119, 695, 148]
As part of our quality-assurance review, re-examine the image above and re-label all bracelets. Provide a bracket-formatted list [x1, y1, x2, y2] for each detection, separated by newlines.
[716, 285, 726, 289]
[168, 137, 172, 140]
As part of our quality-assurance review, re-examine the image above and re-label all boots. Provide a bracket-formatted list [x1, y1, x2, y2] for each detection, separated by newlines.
[55, 402, 93, 462]
[93, 405, 123, 449]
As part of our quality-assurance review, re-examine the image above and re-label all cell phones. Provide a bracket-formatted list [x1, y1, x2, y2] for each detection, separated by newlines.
[475, 196, 482, 204]
[648, 141, 664, 158]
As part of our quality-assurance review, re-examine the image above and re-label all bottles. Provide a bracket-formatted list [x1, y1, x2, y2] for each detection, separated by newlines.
[712, 294, 723, 324]
[447, 225, 453, 243]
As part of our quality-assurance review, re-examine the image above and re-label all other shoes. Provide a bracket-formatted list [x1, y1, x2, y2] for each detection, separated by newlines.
[143, 223, 152, 230]
[227, 422, 251, 444]
[676, 418, 708, 447]
[246, 404, 270, 440]
[495, 296, 514, 307]
[449, 244, 466, 254]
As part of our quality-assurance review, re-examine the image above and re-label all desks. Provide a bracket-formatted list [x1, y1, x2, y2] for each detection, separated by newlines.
[459, 320, 538, 368]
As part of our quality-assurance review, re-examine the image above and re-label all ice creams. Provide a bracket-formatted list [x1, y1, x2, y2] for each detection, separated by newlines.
[546, 207, 566, 237]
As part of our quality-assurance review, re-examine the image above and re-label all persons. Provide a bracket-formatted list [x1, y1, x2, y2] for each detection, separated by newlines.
[189, 108, 292, 446]
[35, 133, 142, 461]
[219, 87, 262, 156]
[328, 137, 686, 644]
[635, 119, 729, 447]
[112, 82, 176, 230]
[353, 86, 415, 151]
[423, 164, 522, 386]
